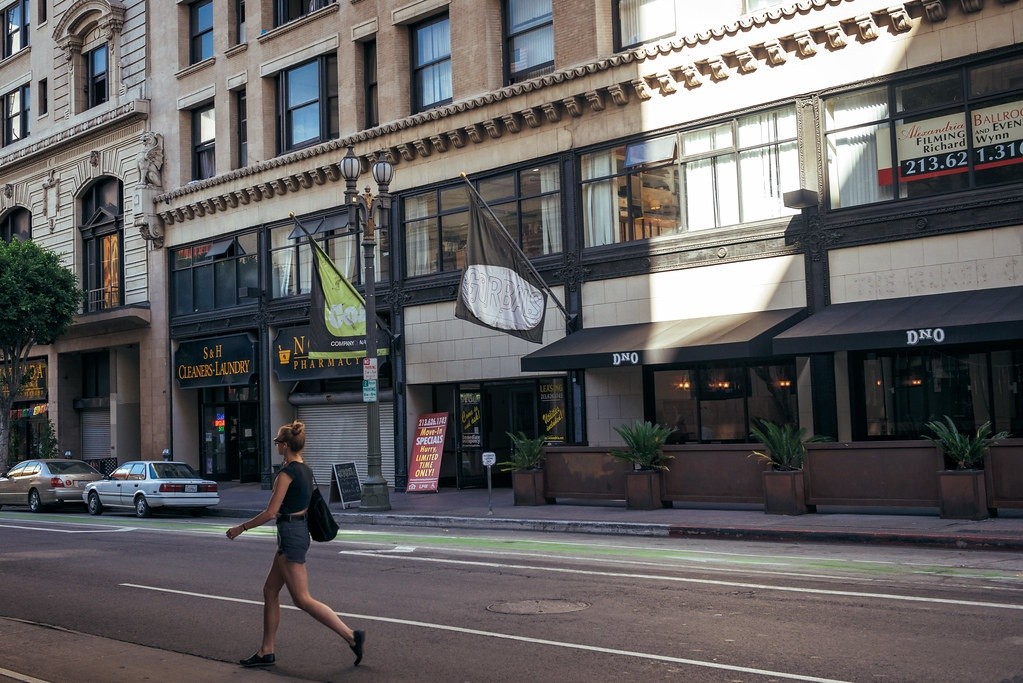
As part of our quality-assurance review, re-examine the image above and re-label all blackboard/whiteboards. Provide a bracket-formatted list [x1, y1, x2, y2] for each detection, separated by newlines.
[328, 461, 361, 502]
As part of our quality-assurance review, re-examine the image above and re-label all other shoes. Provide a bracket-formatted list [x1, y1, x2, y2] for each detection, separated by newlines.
[240, 651, 275, 666]
[350, 630, 366, 666]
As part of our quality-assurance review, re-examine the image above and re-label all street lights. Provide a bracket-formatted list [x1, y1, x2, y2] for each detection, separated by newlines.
[336, 143, 395, 511]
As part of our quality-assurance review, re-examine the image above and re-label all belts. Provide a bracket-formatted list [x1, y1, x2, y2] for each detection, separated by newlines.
[278, 514, 306, 522]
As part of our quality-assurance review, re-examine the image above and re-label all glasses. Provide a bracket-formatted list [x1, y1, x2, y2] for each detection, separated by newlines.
[273, 438, 285, 446]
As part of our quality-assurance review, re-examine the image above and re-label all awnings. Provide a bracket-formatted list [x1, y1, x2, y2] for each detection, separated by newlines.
[521, 307, 807, 372]
[772, 286, 1023, 355]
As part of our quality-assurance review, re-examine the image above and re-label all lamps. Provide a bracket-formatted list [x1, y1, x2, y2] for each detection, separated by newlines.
[239, 288, 259, 299]
[783, 190, 819, 211]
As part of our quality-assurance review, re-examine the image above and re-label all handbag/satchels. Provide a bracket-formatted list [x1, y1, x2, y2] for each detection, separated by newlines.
[307, 473, 339, 542]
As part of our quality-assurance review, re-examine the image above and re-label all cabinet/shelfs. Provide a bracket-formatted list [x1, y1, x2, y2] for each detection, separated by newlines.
[615, 154, 679, 228]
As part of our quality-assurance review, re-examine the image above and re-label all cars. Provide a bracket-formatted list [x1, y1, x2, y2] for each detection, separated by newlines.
[84, 460, 219, 518]
[0, 459, 107, 513]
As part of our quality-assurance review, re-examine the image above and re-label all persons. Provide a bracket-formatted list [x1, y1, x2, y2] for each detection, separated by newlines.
[225, 421, 366, 666]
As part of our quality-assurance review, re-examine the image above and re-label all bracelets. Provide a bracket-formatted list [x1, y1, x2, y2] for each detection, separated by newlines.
[242, 524, 247, 531]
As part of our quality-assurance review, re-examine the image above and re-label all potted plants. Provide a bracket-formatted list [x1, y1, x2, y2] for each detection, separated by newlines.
[497, 430, 557, 506]
[608, 420, 676, 512]
[921, 415, 1014, 521]
[747, 419, 838, 517]
